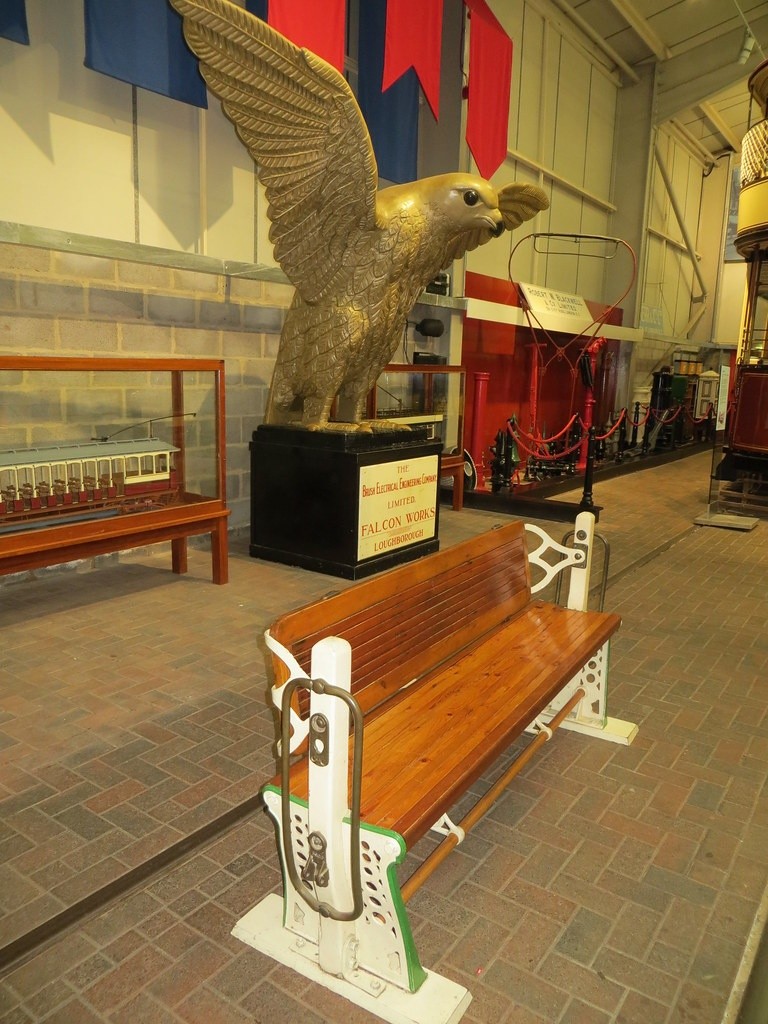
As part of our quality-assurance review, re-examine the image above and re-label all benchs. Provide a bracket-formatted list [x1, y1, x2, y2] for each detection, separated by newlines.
[225, 511, 652, 1024]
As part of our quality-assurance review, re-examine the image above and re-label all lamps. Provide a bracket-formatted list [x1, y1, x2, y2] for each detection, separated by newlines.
[733, 0, 767, 66]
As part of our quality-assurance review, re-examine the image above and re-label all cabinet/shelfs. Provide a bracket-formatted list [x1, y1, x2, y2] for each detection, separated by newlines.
[0, 355, 230, 586]
[329, 363, 468, 511]
